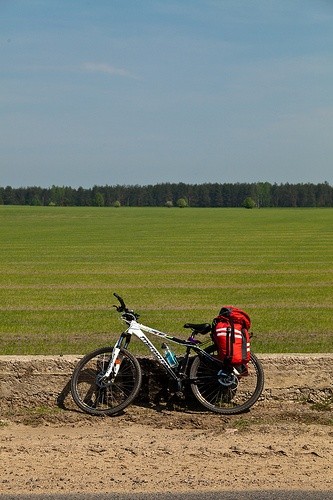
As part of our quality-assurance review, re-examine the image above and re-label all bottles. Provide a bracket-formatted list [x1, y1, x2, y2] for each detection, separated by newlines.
[160, 341, 179, 368]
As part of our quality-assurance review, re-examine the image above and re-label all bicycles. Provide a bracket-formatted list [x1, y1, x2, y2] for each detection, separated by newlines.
[68, 293, 265, 416]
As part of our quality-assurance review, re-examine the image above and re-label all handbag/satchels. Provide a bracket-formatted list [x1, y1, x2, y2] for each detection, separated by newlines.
[212, 306, 253, 365]
